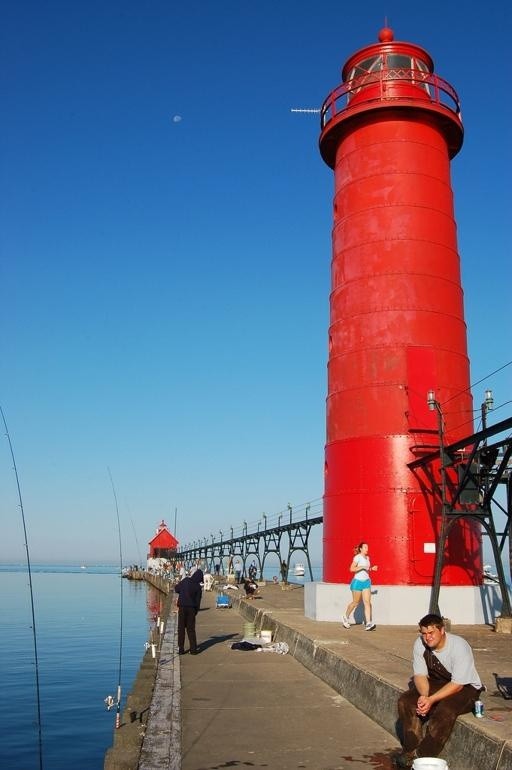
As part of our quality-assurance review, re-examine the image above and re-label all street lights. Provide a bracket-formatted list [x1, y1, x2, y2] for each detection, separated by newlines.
[427, 389, 446, 614]
[481, 388, 512, 617]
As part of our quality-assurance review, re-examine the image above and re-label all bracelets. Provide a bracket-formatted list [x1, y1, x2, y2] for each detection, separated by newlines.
[370, 567, 373, 571]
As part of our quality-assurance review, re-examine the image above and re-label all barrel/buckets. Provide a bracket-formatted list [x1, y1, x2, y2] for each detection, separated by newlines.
[412, 757, 449, 770]
[226, 574, 235, 583]
[243, 624, 256, 638]
[261, 630, 272, 642]
[218, 596, 229, 604]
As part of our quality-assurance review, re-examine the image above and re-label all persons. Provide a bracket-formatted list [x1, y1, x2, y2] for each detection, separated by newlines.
[394, 614, 485, 770]
[342, 542, 379, 631]
[174, 568, 204, 655]
[279, 558, 287, 584]
[120, 556, 259, 601]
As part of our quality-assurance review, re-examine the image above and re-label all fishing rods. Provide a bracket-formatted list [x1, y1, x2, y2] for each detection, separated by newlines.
[127, 501, 157, 659]
[105, 467, 123, 730]
[0, 408, 43, 770]
[173, 509, 177, 574]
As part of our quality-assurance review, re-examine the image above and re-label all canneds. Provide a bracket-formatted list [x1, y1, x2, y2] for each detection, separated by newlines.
[474, 701, 485, 719]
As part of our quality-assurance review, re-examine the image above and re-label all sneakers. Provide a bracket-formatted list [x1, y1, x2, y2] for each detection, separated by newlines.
[362, 623, 376, 631]
[177, 649, 198, 655]
[342, 617, 351, 630]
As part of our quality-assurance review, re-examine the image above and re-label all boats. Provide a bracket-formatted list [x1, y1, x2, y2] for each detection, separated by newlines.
[294, 564, 304, 576]
[483, 565, 499, 584]
